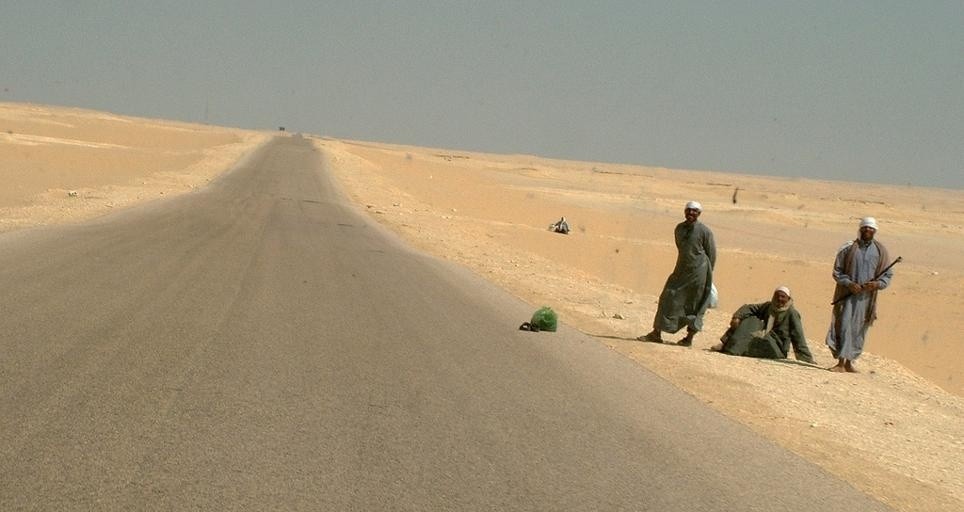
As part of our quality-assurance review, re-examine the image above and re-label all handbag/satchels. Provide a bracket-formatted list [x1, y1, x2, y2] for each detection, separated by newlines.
[706, 280, 718, 309]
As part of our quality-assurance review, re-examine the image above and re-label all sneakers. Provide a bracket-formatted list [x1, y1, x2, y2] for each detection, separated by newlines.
[829, 364, 861, 373]
[636, 329, 664, 343]
[677, 336, 693, 347]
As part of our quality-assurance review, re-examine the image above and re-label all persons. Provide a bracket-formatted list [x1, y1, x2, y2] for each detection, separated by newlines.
[825, 216, 894, 373]
[633, 200, 716, 347]
[550, 216, 570, 235]
[710, 284, 817, 365]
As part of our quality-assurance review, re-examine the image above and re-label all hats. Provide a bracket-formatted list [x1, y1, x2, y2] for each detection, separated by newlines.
[685, 201, 703, 212]
[858, 216, 879, 232]
[774, 285, 793, 299]
